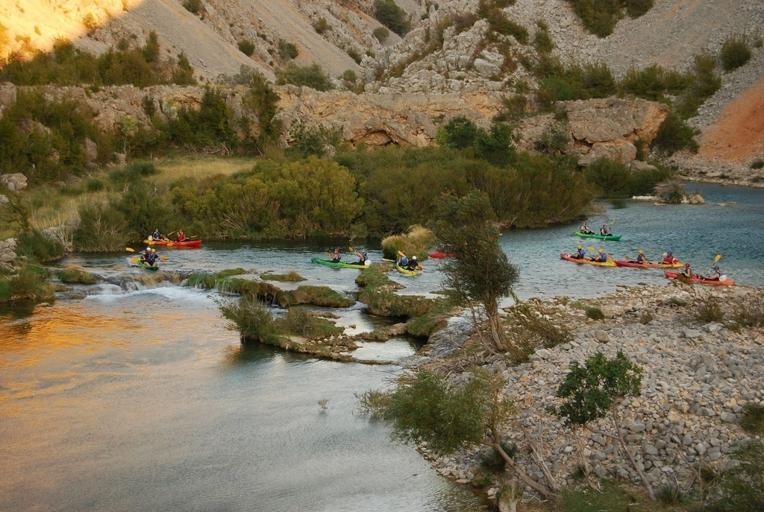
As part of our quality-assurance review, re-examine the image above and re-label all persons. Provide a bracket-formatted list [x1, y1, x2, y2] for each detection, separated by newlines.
[152, 227, 164, 241]
[174, 229, 186, 243]
[699, 264, 721, 281]
[405, 255, 423, 272]
[140, 248, 161, 264]
[599, 224, 613, 236]
[346, 248, 368, 266]
[579, 221, 596, 235]
[142, 247, 158, 267]
[569, 245, 587, 259]
[628, 249, 646, 264]
[586, 247, 608, 262]
[396, 249, 409, 266]
[331, 246, 342, 263]
[678, 263, 693, 278]
[657, 250, 673, 265]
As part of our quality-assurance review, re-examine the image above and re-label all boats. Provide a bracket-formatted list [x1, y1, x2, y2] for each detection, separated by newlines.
[665, 271, 735, 285]
[615, 260, 683, 268]
[144, 238, 202, 248]
[560, 252, 616, 267]
[383, 258, 418, 276]
[312, 257, 370, 269]
[575, 231, 621, 241]
[432, 252, 458, 258]
[139, 256, 159, 270]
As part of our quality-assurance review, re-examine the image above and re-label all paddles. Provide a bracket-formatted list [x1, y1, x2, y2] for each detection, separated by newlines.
[638, 249, 651, 264]
[588, 246, 594, 256]
[662, 252, 668, 256]
[598, 248, 604, 253]
[712, 254, 721, 266]
[348, 246, 364, 261]
[125, 247, 169, 261]
[577, 244, 583, 251]
[602, 234, 607, 243]
[131, 257, 156, 262]
[398, 251, 422, 270]
